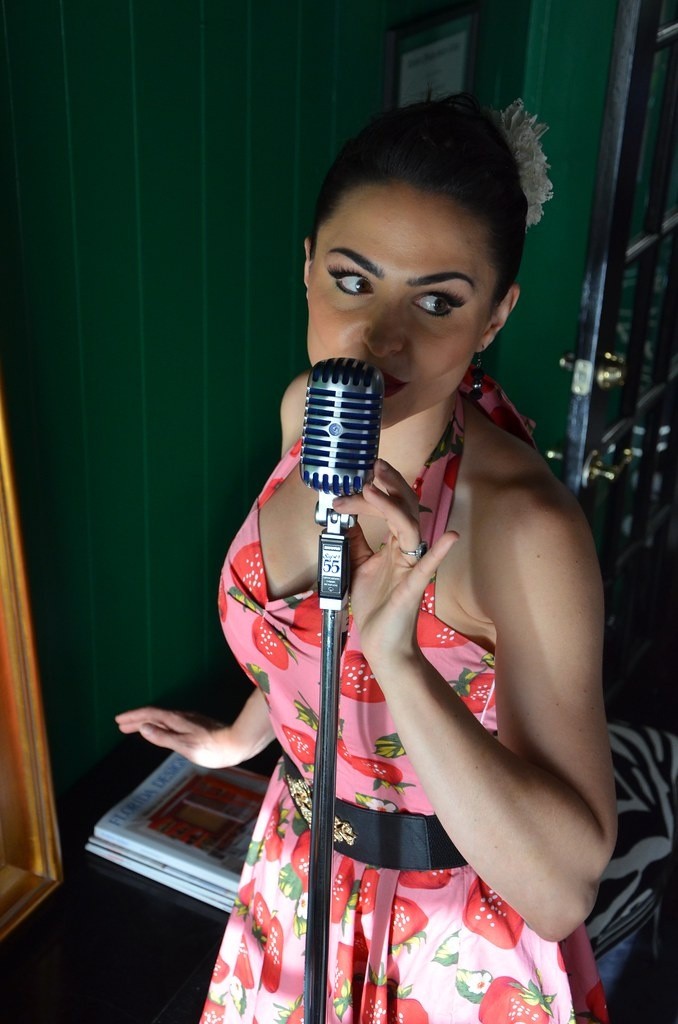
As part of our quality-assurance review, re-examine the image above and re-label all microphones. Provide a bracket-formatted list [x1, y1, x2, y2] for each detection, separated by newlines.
[298, 357, 384, 631]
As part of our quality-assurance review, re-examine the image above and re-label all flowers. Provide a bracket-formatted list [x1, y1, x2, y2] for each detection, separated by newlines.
[500, 97, 555, 232]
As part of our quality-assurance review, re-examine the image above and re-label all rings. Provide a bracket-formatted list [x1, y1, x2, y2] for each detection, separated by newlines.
[400, 541, 427, 560]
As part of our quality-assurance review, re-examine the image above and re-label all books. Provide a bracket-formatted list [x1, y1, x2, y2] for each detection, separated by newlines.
[85, 748, 276, 914]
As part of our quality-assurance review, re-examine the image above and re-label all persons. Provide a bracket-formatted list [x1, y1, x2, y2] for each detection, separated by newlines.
[114, 96, 617, 1024]
